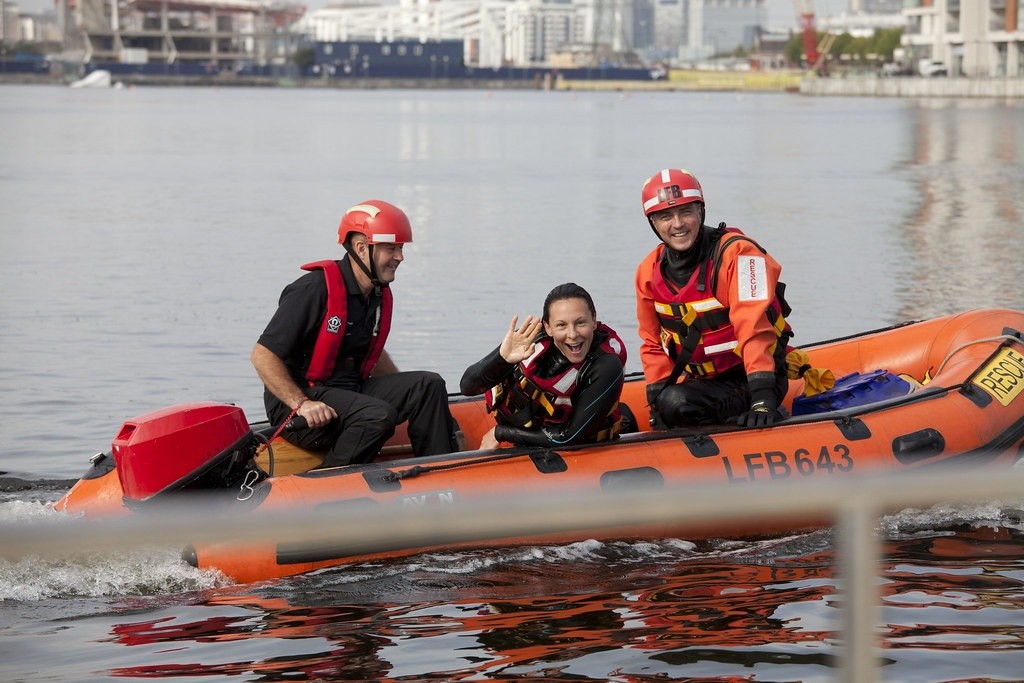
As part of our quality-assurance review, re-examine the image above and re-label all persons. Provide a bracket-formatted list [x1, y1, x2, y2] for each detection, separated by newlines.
[635, 169, 793, 430]
[459, 282, 639, 449]
[250, 199, 466, 467]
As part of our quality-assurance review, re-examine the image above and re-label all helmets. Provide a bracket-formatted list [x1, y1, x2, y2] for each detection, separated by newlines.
[338, 200, 414, 245]
[641, 168, 705, 216]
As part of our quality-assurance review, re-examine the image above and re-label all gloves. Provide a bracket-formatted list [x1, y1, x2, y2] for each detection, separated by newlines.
[650, 410, 668, 430]
[727, 388, 782, 428]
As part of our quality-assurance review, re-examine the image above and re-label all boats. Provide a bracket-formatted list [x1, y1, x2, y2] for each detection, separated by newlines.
[53, 308, 1024, 583]
